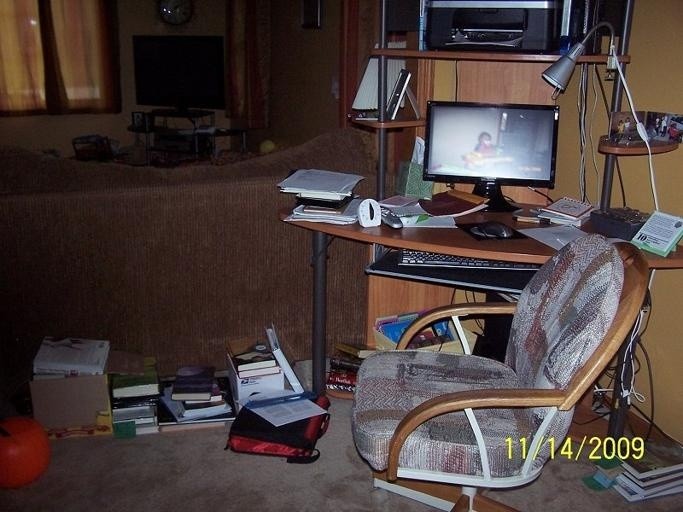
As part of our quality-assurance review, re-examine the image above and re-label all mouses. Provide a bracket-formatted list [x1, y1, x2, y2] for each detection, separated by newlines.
[470, 221, 514, 239]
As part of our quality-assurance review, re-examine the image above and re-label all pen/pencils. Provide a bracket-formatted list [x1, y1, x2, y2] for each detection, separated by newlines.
[513, 215, 552, 225]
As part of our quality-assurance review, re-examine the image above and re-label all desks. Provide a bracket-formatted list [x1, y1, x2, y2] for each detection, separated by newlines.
[278, 194, 682, 456]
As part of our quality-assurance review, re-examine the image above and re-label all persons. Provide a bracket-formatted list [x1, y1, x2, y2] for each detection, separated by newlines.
[610, 112, 682, 144]
[473, 133, 495, 155]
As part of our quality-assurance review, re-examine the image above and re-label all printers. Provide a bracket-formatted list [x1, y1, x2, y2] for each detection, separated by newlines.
[426, 0, 564, 55]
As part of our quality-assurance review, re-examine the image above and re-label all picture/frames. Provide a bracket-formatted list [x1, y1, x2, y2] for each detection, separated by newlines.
[131, 111, 144, 129]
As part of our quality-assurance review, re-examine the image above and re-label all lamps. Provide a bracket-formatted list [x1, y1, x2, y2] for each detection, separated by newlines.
[540, 21, 618, 99]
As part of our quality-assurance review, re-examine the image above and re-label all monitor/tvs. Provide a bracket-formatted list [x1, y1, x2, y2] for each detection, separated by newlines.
[132, 35, 226, 118]
[422, 100, 560, 212]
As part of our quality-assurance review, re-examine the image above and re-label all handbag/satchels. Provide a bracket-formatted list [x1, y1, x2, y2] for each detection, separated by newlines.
[225, 392, 331, 464]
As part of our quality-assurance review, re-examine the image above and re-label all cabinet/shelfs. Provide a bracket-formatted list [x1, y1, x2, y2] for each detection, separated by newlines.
[349, 1, 679, 157]
[126, 108, 247, 167]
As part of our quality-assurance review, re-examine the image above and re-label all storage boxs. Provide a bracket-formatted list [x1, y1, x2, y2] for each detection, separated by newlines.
[372, 318, 478, 357]
[0, 349, 146, 439]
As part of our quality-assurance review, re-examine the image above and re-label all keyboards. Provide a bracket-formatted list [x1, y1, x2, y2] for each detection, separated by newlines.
[397, 248, 543, 271]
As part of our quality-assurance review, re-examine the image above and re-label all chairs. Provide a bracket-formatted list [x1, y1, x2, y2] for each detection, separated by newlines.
[353, 231, 650, 511]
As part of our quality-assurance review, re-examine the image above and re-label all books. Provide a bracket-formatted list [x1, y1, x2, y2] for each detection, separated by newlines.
[538, 196, 595, 227]
[277, 165, 490, 228]
[630, 210, 682, 258]
[584, 434, 683, 504]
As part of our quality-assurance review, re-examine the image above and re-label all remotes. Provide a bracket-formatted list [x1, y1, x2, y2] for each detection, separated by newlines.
[380, 205, 403, 229]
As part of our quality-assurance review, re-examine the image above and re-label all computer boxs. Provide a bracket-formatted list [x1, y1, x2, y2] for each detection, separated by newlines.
[482, 291, 521, 364]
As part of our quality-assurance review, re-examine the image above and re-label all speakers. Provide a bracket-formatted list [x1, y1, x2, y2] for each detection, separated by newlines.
[300, 0, 322, 29]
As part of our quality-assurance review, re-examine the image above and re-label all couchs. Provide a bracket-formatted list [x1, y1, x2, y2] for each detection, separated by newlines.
[0, 127, 394, 409]
